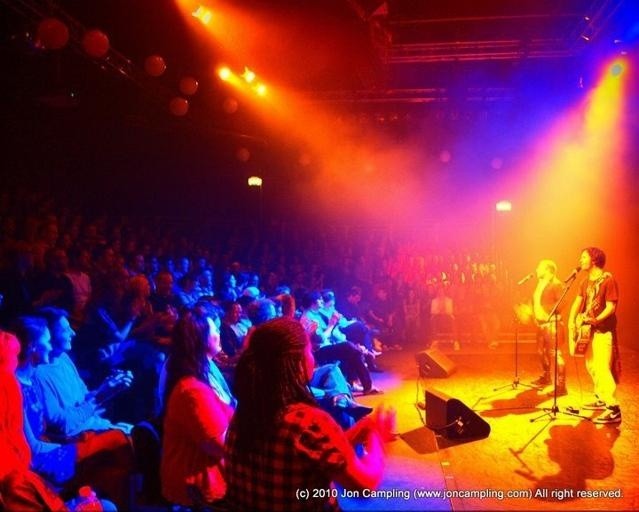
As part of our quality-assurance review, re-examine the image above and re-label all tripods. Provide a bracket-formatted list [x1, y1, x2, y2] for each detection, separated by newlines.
[530, 327, 591, 423]
[493, 339, 542, 391]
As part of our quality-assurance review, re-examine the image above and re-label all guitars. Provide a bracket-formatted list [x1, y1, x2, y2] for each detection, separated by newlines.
[568, 287, 597, 355]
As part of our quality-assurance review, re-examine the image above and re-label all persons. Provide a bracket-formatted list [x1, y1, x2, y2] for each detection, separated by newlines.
[569, 247, 623, 425]
[1, 197, 514, 511]
[531, 259, 567, 397]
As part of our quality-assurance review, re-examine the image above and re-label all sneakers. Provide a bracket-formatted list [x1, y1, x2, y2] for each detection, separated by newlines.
[582, 400, 607, 410]
[548, 388, 567, 397]
[592, 409, 621, 424]
[363, 388, 384, 395]
[365, 349, 384, 373]
[531, 377, 552, 385]
[351, 384, 364, 392]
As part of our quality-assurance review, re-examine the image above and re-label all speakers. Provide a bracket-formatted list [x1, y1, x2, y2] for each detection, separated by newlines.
[424, 389, 490, 441]
[416, 349, 456, 377]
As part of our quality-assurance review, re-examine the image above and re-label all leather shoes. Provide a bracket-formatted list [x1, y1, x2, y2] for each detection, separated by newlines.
[336, 398, 373, 415]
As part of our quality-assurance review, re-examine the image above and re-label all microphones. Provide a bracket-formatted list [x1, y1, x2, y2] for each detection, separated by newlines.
[518, 273, 533, 286]
[564, 267, 581, 283]
